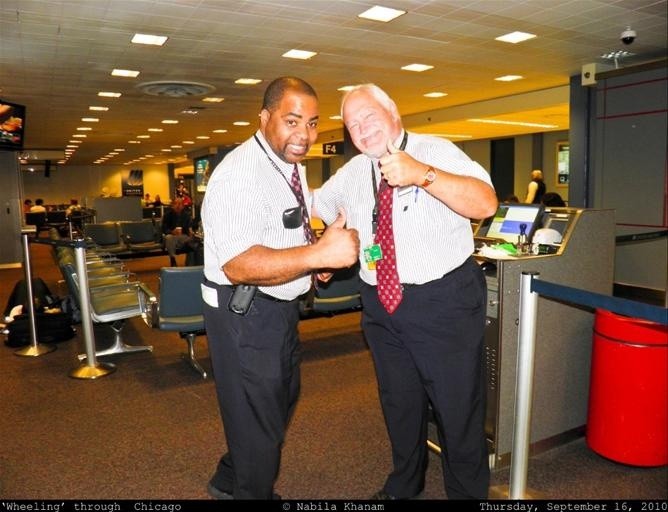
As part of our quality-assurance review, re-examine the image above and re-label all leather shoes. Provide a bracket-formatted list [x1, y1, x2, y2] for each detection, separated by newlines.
[371, 490, 396, 501]
[207, 478, 281, 501]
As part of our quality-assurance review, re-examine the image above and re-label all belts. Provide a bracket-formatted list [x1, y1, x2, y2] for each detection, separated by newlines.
[201, 274, 298, 302]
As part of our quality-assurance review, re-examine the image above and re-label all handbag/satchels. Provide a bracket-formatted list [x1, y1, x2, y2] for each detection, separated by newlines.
[3, 277, 75, 347]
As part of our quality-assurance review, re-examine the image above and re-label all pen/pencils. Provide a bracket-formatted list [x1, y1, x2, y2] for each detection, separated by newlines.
[415, 185, 419, 203]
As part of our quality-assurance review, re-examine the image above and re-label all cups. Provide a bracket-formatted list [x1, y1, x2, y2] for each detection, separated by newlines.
[176, 227, 182, 240]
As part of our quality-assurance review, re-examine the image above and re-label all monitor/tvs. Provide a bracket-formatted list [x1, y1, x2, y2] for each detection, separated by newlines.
[193, 154, 225, 193]
[0, 99, 27, 153]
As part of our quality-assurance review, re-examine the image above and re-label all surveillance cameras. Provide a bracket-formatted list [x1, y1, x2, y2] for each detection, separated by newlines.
[619, 30, 638, 46]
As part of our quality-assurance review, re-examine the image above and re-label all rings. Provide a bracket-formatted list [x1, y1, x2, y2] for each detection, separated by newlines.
[386, 172, 393, 179]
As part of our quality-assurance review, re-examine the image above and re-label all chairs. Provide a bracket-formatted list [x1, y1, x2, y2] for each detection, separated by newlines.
[25, 203, 365, 379]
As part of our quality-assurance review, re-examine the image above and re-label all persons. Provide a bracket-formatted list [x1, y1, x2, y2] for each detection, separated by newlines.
[307, 82, 500, 500]
[65, 198, 81, 216]
[522, 169, 546, 204]
[542, 191, 565, 206]
[503, 194, 518, 204]
[23, 198, 33, 212]
[204, 74, 360, 504]
[29, 198, 47, 217]
[141, 193, 152, 205]
[181, 191, 192, 206]
[160, 196, 198, 267]
[145, 194, 163, 207]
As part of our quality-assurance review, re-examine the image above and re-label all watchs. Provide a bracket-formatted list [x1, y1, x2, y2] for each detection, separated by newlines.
[421, 164, 437, 188]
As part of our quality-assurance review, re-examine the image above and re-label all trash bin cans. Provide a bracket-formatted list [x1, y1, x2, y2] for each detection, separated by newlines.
[586, 307, 668, 469]
[49, 223, 67, 240]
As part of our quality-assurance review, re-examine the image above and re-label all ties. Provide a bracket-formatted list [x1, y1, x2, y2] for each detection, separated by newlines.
[373, 160, 404, 314]
[292, 162, 320, 298]
